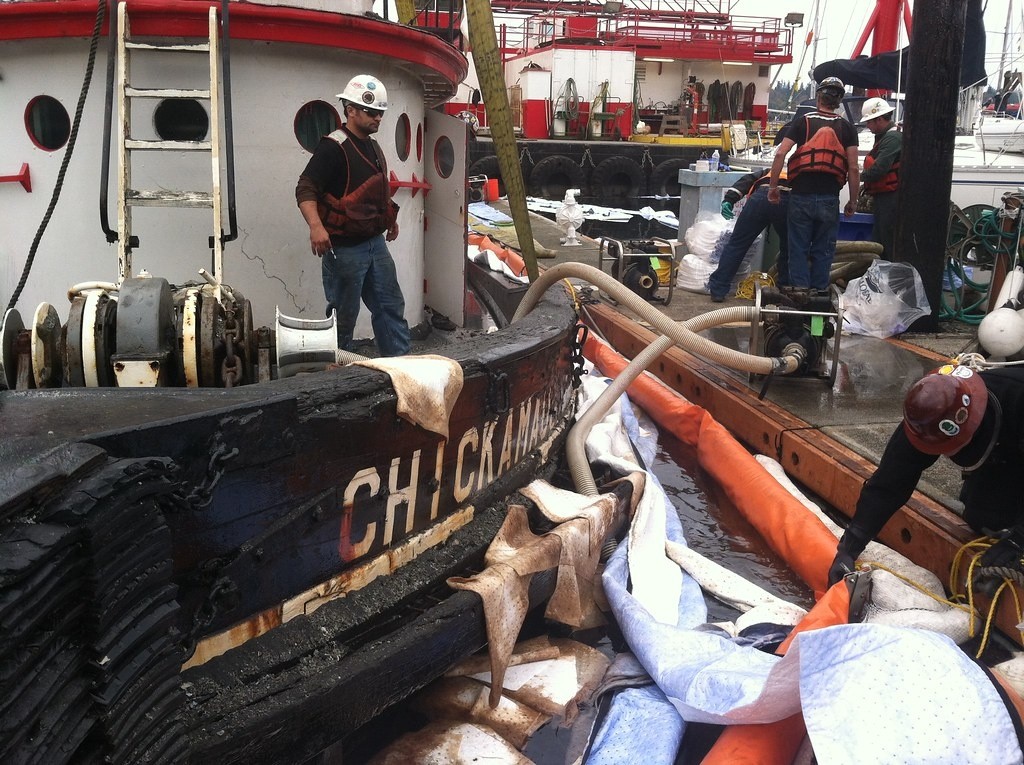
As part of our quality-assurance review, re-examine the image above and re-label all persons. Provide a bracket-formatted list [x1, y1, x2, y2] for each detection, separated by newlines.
[767, 76, 859, 291]
[296, 73, 412, 357]
[827, 365, 1024, 589]
[860, 97, 903, 262]
[709, 169, 787, 302]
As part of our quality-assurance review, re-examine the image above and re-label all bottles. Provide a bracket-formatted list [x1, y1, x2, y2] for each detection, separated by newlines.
[711, 150, 720, 171]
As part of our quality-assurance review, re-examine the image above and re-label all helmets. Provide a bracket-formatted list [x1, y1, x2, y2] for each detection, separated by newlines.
[335, 75, 388, 110]
[816, 76, 845, 98]
[859, 97, 896, 123]
[903, 366, 988, 456]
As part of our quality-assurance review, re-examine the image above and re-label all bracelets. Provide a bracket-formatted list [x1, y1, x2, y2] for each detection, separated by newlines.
[769, 188, 776, 190]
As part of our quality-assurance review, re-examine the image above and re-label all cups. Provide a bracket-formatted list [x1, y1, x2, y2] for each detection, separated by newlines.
[696, 160, 709, 171]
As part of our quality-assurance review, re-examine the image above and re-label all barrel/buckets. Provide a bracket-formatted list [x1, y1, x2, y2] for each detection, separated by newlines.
[484, 179, 499, 201]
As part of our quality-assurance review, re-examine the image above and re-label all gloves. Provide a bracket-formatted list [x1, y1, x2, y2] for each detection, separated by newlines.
[980, 532, 1023, 568]
[721, 201, 735, 220]
[827, 550, 855, 589]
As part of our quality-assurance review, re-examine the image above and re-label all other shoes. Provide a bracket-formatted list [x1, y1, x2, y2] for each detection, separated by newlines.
[710, 295, 723, 302]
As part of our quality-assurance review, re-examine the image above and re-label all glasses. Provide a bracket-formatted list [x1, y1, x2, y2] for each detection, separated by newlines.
[350, 103, 384, 118]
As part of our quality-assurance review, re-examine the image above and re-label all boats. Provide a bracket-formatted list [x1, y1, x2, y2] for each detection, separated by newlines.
[0, 1, 638, 765]
[366, 0, 808, 219]
[726, 0, 1024, 242]
